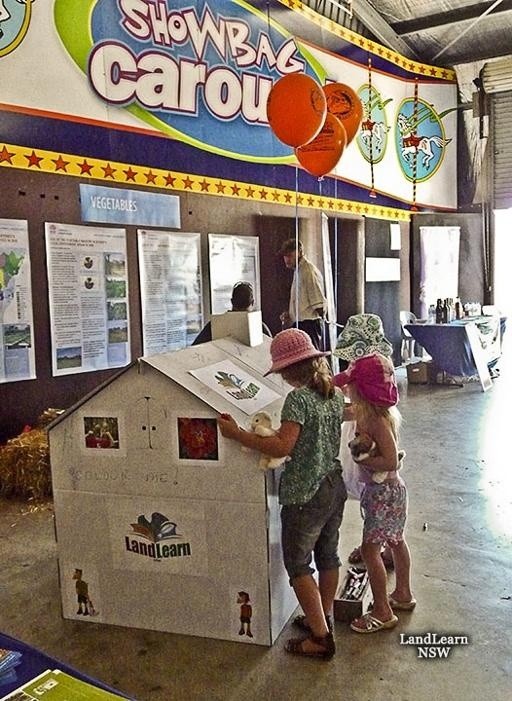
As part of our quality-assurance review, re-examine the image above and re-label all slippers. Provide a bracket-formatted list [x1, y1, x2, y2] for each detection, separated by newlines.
[350, 612, 399, 634]
[371, 592, 416, 609]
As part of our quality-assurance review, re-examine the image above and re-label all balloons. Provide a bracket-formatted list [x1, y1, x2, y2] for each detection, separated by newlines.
[266, 73, 363, 178]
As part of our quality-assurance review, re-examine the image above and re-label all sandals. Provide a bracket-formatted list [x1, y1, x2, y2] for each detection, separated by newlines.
[294, 614, 334, 634]
[284, 633, 335, 660]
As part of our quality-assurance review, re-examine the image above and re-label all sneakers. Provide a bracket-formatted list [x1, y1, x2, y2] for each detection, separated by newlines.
[348, 546, 394, 569]
[77, 610, 89, 616]
[239, 629, 253, 638]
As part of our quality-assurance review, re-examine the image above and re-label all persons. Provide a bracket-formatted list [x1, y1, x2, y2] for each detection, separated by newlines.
[217, 314, 415, 657]
[190, 238, 328, 361]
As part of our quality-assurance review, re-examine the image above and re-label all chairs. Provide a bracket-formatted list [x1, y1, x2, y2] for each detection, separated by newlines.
[399, 311, 422, 362]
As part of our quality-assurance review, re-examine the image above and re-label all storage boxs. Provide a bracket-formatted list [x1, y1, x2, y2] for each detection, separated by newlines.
[405, 360, 431, 384]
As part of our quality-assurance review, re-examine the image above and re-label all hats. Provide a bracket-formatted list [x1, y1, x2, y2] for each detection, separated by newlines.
[278, 238, 302, 255]
[351, 352, 399, 408]
[232, 279, 255, 307]
[333, 312, 394, 363]
[262, 326, 331, 378]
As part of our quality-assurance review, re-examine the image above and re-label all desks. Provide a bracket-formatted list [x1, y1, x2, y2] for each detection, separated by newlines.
[405, 316, 507, 389]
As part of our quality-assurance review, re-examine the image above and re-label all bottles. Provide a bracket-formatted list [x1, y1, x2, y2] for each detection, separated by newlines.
[427, 298, 459, 323]
